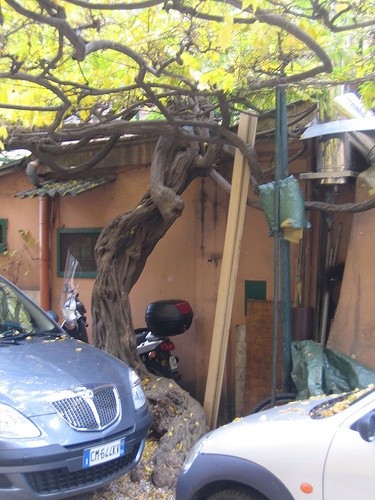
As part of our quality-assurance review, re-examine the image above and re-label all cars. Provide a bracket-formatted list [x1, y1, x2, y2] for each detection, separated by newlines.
[0, 275, 153, 500]
[175, 383, 375, 500]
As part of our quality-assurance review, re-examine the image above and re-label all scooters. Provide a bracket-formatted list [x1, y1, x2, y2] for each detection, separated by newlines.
[56, 246, 194, 381]
